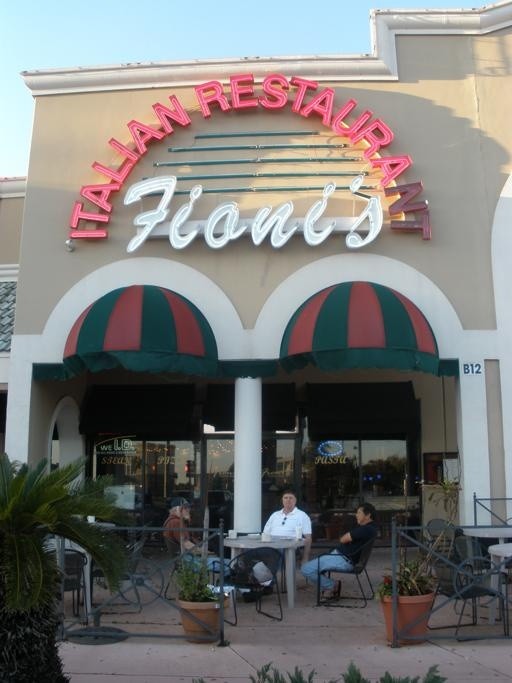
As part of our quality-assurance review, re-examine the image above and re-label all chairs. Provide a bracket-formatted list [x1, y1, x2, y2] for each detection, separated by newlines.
[314, 495, 420, 545]
[283, 548, 309, 592]
[44, 549, 88, 626]
[162, 537, 211, 600]
[88, 541, 142, 615]
[425, 517, 509, 641]
[212, 547, 284, 626]
[316, 534, 376, 609]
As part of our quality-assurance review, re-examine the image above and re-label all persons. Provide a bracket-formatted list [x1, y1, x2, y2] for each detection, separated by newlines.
[243, 491, 313, 602]
[163, 496, 233, 600]
[300, 502, 377, 601]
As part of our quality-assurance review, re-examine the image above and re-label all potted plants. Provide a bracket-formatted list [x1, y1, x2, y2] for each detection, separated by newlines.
[169, 546, 231, 644]
[416, 446, 462, 525]
[375, 549, 440, 645]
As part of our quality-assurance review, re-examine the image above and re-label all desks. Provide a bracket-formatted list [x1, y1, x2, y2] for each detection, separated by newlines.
[487, 543, 512, 591]
[223, 536, 307, 610]
[462, 527, 510, 567]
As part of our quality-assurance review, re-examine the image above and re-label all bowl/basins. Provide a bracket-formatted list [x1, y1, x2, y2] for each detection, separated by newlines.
[248, 534, 261, 539]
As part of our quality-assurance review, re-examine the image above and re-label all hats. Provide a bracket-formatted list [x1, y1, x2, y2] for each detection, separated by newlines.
[168, 495, 194, 507]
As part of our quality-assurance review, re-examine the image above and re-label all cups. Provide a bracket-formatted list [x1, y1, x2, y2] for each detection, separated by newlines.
[261, 533, 272, 541]
[295, 527, 303, 539]
[229, 530, 237, 538]
[87, 515, 95, 523]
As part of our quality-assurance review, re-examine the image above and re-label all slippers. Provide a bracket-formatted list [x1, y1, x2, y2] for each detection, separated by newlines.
[333, 579, 341, 600]
